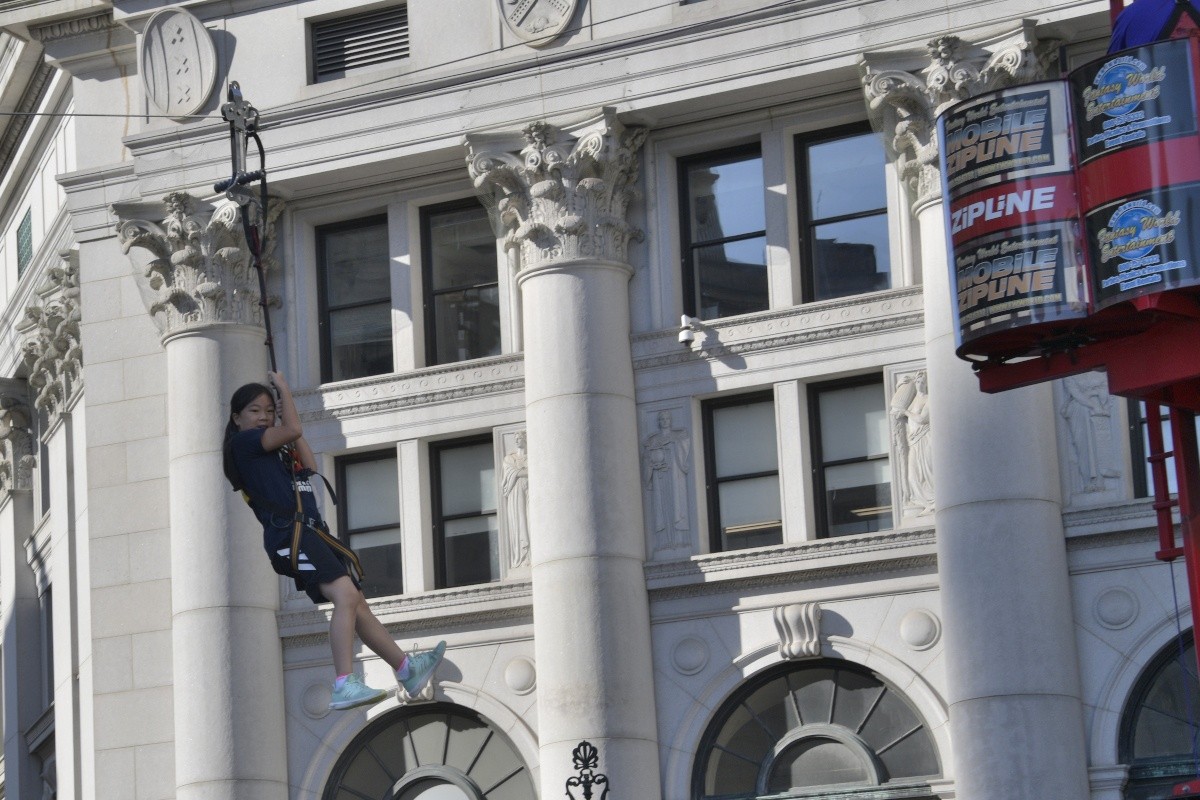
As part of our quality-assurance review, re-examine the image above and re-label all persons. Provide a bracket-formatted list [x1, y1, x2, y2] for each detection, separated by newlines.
[221, 371, 446, 709]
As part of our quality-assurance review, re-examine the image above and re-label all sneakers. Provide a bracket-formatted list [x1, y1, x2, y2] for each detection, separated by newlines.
[395, 640, 447, 698]
[327, 672, 387, 711]
